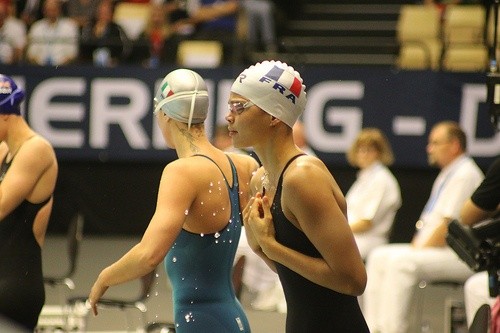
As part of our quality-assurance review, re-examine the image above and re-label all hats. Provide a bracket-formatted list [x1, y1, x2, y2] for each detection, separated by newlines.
[232, 61, 307, 128]
[155, 68, 210, 124]
[0, 74, 24, 111]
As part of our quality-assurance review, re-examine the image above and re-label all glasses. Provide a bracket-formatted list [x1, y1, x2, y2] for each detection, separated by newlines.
[226, 101, 256, 115]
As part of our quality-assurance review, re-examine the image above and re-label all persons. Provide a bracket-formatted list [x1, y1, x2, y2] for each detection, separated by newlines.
[211, 120, 500, 333]
[0, 0, 249, 71]
[232, 114, 318, 313]
[88, 69, 260, 333]
[225, 60, 370, 333]
[213, 124, 244, 153]
[0, 73, 58, 333]
